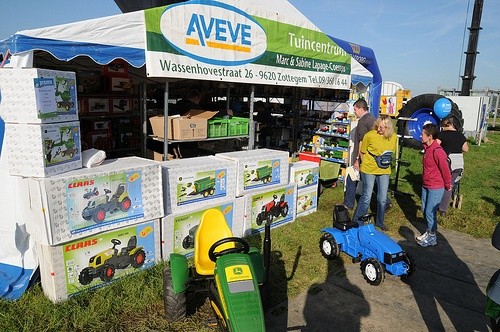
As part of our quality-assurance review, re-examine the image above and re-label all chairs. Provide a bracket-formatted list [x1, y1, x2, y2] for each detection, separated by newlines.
[193, 208, 236, 277]
[332, 204, 359, 232]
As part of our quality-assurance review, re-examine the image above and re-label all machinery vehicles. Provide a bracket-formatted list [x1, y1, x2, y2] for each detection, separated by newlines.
[319, 205, 416, 286]
[163, 209, 266, 332]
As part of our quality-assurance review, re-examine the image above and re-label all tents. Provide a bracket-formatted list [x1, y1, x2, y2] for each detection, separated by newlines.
[0, 0, 350, 301]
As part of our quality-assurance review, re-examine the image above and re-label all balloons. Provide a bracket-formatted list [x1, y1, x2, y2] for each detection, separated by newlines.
[434, 98, 452, 121]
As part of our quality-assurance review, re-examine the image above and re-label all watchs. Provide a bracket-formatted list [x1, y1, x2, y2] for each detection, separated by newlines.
[356, 157, 359, 160]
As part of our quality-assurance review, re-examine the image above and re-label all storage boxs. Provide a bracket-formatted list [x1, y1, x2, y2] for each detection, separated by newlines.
[0, 64, 351, 304]
[380, 95, 396, 115]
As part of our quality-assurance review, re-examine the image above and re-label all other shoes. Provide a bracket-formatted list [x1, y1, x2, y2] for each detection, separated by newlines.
[386, 203, 392, 211]
[375, 224, 388, 232]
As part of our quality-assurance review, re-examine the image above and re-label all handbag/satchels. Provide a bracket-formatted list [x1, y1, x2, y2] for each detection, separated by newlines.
[368, 151, 392, 169]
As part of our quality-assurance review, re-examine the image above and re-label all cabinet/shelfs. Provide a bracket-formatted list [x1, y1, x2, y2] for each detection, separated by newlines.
[210, 89, 295, 157]
[142, 79, 255, 161]
[295, 86, 370, 170]
[77, 81, 143, 154]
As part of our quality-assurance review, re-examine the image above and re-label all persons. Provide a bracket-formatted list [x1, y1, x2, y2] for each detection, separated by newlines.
[484, 222, 500, 332]
[352, 115, 397, 229]
[342, 98, 376, 210]
[415, 124, 452, 247]
[439, 116, 468, 216]
[388, 98, 395, 114]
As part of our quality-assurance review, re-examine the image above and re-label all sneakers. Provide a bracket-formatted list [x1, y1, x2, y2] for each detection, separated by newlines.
[415, 232, 429, 241]
[417, 233, 438, 247]
